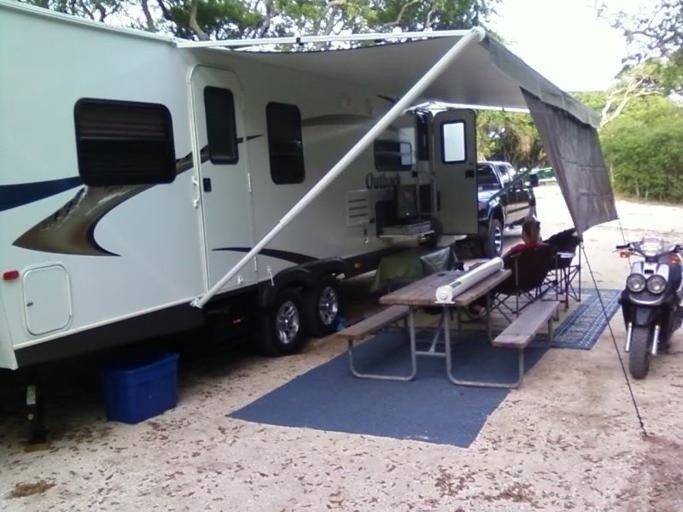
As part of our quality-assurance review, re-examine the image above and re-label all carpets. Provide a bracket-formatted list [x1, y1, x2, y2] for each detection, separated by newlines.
[428, 283, 624, 351]
[223, 325, 549, 447]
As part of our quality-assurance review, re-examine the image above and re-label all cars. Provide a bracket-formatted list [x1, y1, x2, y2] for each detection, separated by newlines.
[470, 158, 542, 261]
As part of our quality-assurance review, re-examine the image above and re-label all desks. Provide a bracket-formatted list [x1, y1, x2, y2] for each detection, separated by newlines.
[378, 266, 512, 384]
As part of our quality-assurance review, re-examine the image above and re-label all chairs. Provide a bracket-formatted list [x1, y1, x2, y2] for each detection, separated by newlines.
[512, 225, 588, 309]
[483, 245, 560, 325]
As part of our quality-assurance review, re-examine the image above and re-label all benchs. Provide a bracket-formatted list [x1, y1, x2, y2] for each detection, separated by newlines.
[336, 304, 410, 379]
[492, 301, 560, 390]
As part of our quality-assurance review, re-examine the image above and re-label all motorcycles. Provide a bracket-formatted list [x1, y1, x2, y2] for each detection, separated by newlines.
[611, 237, 683, 381]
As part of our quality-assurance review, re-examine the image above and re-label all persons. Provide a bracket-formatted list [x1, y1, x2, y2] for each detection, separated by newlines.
[470, 221, 545, 316]
